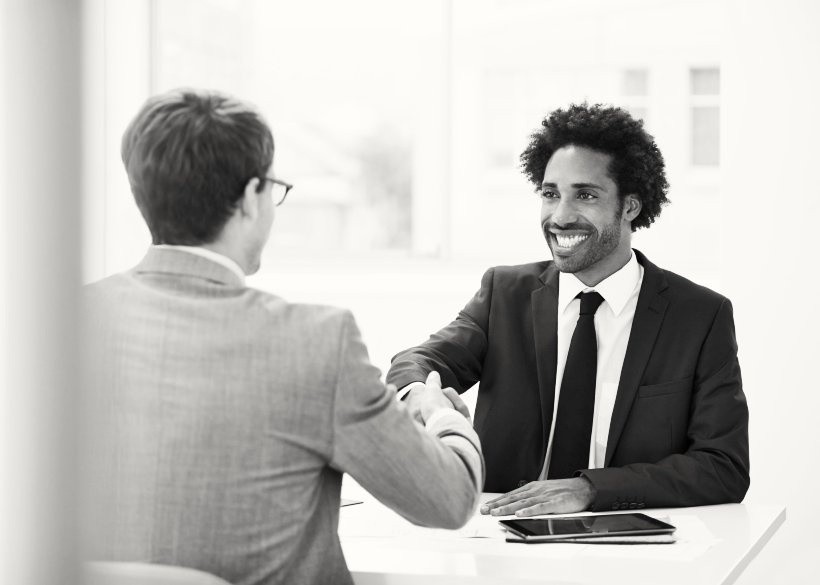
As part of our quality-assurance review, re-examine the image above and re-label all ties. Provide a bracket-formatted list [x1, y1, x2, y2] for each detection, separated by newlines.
[546, 292, 606, 480]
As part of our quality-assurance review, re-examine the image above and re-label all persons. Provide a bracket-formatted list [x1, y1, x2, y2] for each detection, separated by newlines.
[387, 101, 753, 519]
[72, 88, 485, 583]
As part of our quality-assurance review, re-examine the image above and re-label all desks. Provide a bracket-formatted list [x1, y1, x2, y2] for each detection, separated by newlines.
[332, 492, 787, 585]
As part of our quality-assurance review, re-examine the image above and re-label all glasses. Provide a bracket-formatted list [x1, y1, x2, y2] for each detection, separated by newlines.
[265, 176, 293, 207]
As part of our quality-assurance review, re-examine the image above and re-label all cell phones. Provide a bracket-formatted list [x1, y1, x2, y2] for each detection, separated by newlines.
[497, 512, 677, 541]
